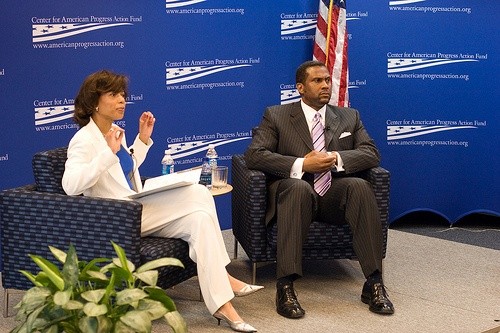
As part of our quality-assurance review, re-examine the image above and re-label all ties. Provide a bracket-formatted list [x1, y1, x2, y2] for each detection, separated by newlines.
[311, 112, 332, 196]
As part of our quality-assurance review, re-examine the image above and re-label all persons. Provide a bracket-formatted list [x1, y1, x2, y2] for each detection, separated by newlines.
[245, 61, 394, 318]
[63, 69, 265, 333]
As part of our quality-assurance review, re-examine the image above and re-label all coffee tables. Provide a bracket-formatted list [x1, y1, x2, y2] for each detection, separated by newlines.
[207, 184, 233, 197]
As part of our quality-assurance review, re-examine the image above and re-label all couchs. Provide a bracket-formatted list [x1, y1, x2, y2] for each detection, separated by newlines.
[0, 146, 204, 320]
[228, 127, 389, 285]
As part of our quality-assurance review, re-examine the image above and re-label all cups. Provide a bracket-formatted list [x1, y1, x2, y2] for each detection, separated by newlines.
[211, 166, 228, 190]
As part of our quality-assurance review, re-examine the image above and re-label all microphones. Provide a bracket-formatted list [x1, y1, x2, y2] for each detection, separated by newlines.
[327, 126, 330, 130]
[130, 148, 134, 153]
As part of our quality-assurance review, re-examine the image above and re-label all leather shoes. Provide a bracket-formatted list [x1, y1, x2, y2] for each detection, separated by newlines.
[275, 283, 304, 318]
[360, 281, 394, 313]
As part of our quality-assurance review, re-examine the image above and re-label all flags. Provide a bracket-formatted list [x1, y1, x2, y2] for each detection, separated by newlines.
[315, 0, 351, 107]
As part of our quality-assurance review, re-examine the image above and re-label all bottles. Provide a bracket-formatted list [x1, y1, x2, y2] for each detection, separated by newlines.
[205, 144, 218, 167]
[159, 150, 174, 175]
[198, 156, 212, 190]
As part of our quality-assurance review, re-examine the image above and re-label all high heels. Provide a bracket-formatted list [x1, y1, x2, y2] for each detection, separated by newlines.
[236, 284, 265, 296]
[213, 310, 258, 332]
